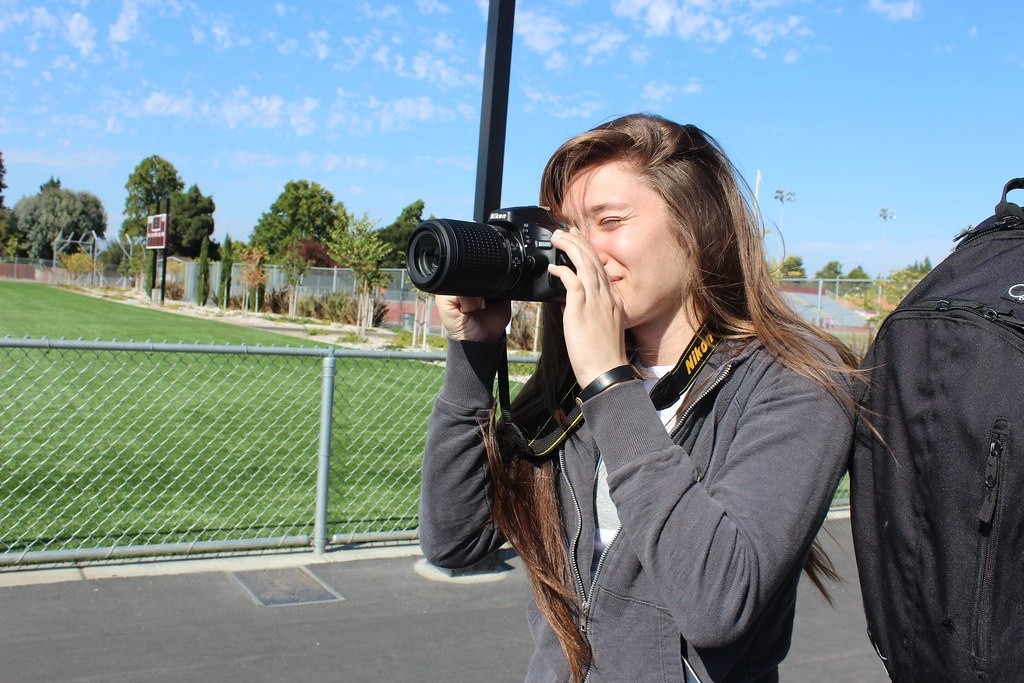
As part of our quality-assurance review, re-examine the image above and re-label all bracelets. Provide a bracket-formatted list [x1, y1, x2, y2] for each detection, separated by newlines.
[573, 364, 644, 411]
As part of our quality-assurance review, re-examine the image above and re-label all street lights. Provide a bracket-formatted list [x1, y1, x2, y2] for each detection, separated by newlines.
[877, 206, 897, 316]
[774, 189, 797, 255]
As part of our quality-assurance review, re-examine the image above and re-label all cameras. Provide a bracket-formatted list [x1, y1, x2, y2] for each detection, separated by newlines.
[407, 205, 578, 304]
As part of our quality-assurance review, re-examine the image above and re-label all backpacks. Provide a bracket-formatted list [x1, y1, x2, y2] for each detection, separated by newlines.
[847, 177, 1024, 683]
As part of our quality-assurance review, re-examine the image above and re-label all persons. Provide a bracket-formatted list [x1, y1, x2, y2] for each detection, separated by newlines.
[417, 113, 859, 683]
